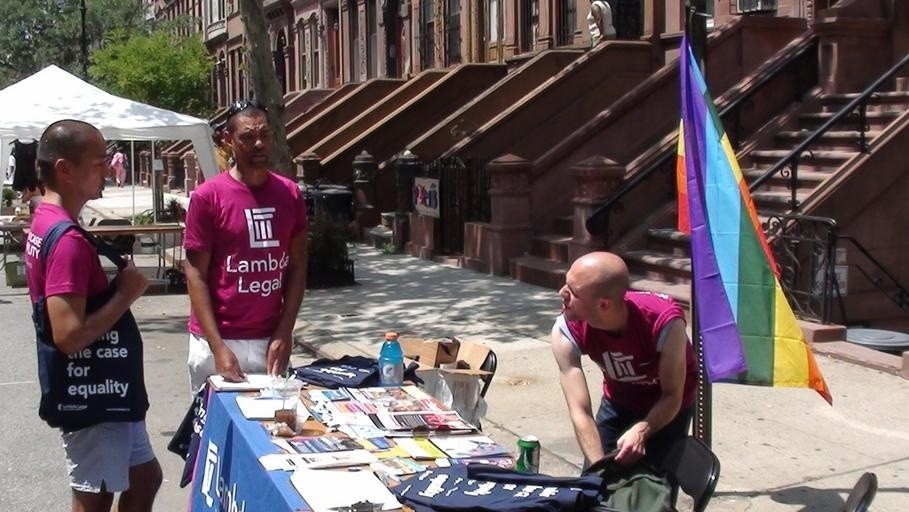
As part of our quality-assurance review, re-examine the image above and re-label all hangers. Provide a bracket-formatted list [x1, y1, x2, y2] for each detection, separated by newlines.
[8, 133, 40, 145]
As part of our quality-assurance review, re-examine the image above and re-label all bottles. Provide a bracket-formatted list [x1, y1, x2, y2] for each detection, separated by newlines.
[377, 331, 405, 387]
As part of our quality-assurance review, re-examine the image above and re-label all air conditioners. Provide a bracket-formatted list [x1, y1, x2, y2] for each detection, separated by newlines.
[735, 0, 777, 15]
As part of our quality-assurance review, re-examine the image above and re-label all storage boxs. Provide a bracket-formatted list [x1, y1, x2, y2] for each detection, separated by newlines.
[5, 261, 28, 289]
[368, 330, 493, 417]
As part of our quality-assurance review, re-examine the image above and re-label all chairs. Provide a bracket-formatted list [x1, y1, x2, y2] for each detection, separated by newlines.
[586, 432, 721, 512]
[470, 347, 497, 434]
[840, 470, 879, 512]
[92, 218, 135, 267]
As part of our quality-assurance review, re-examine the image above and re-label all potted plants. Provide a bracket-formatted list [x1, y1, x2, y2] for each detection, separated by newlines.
[1, 188, 18, 217]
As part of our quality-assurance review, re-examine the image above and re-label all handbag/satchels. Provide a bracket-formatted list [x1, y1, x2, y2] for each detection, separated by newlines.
[31, 218, 151, 430]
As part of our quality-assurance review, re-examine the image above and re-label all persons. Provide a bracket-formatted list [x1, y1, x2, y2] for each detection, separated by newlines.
[110, 147, 128, 188]
[24, 121, 163, 512]
[4, 149, 16, 190]
[551, 251, 697, 480]
[183, 101, 308, 391]
[588, 1, 617, 48]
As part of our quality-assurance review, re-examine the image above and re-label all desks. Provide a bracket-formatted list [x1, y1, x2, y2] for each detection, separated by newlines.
[169, 359, 623, 512]
[76, 222, 186, 296]
[0, 221, 33, 272]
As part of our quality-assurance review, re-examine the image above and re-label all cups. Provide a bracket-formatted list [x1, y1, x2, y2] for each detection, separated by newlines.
[272, 378, 302, 436]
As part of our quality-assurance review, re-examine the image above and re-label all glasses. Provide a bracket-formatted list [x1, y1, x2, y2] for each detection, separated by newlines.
[224, 99, 268, 118]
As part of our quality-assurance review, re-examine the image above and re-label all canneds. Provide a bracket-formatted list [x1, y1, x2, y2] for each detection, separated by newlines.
[515, 436, 540, 475]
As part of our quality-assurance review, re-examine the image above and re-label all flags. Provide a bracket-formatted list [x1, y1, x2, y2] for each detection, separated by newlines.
[676, 34, 834, 407]
